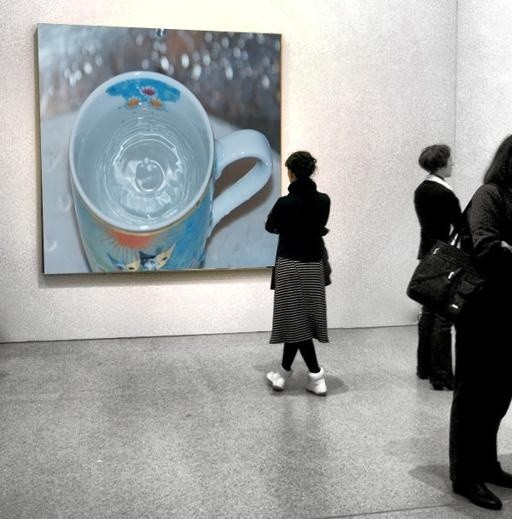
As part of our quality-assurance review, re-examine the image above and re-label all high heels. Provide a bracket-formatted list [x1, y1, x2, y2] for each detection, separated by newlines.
[417, 367, 455, 390]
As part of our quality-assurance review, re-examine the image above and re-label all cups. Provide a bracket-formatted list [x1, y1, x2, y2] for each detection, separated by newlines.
[66, 69, 273, 274]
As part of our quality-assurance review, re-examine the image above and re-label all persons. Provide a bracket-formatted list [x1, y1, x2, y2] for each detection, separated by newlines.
[264, 151, 332, 395]
[406, 135, 512, 510]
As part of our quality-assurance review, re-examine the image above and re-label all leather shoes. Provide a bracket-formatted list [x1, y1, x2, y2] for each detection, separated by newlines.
[452, 462, 512, 509]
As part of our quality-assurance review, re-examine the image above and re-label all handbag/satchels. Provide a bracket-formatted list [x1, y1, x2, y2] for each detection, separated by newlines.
[407, 240, 489, 319]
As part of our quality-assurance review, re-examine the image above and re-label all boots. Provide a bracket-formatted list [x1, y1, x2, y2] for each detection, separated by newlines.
[304, 368, 327, 395]
[266, 365, 292, 390]
